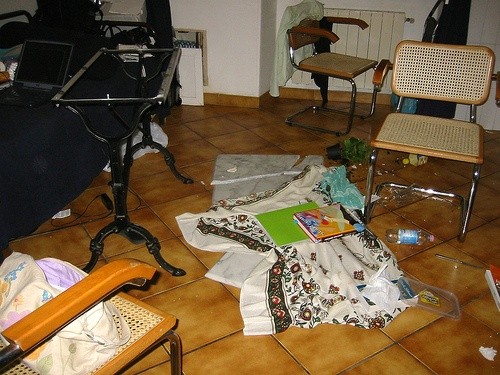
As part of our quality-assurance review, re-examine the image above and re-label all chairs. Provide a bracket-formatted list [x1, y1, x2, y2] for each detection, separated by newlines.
[1, 257, 183, 374]
[1, 10, 35, 49]
[277, 0, 380, 137]
[364, 40, 499, 242]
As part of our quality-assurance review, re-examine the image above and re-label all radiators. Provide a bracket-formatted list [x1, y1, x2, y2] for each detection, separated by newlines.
[288, 8, 415, 91]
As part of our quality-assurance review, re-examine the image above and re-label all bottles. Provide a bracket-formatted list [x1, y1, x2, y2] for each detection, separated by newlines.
[387, 228, 434, 247]
[403, 154, 429, 167]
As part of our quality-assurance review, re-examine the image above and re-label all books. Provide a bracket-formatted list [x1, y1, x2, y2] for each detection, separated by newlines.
[116, 44, 155, 63]
[291, 204, 367, 243]
[254, 200, 323, 248]
[484, 264, 500, 313]
